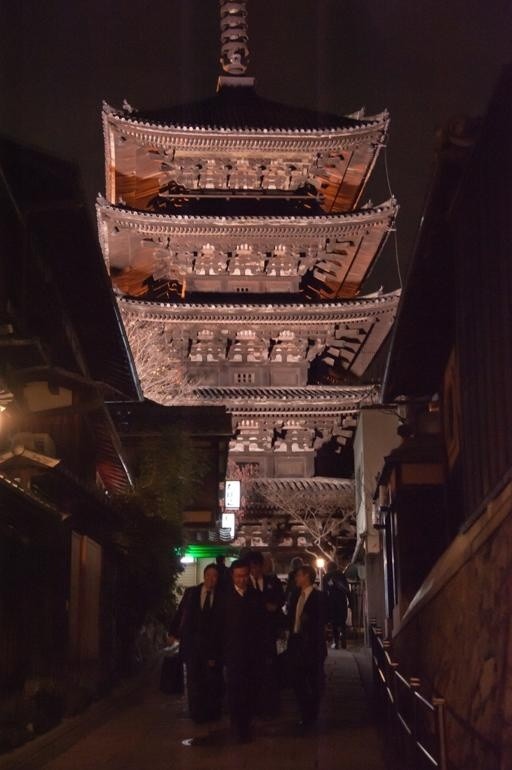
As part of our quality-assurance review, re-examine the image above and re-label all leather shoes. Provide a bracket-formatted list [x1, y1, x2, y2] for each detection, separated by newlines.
[330, 644, 347, 648]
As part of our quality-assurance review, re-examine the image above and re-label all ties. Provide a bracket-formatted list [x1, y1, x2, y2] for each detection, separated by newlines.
[202, 591, 211, 612]
[254, 576, 261, 592]
[296, 591, 306, 631]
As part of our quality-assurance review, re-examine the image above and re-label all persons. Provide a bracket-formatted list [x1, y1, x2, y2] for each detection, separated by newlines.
[284, 555, 305, 606]
[321, 561, 355, 650]
[165, 559, 227, 727]
[206, 560, 278, 748]
[266, 565, 338, 736]
[239, 549, 286, 610]
